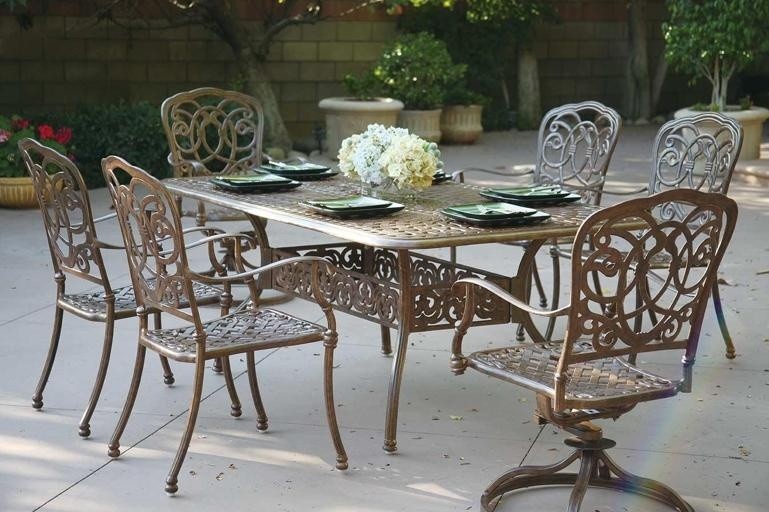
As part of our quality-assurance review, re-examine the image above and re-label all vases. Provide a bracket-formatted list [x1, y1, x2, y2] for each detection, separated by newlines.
[357, 175, 415, 206]
[0, 173, 62, 208]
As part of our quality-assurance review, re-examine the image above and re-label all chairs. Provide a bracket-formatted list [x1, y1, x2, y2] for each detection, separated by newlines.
[450, 188, 739, 512]
[545, 110, 744, 369]
[22, 136, 259, 439]
[450, 101, 621, 341]
[160, 86, 266, 315]
[101, 154, 348, 494]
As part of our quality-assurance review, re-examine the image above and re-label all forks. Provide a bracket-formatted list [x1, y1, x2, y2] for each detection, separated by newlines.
[475, 203, 516, 215]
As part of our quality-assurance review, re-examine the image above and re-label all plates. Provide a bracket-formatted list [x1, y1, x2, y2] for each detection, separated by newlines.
[439, 183, 582, 228]
[207, 162, 339, 194]
[299, 195, 406, 217]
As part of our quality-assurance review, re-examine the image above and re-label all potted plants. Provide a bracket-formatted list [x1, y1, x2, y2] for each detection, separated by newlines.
[319, 32, 483, 161]
[660, 2, 768, 163]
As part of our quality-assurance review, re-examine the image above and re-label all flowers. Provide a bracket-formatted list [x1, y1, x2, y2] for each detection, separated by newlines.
[337, 123, 444, 194]
[0, 114, 78, 179]
